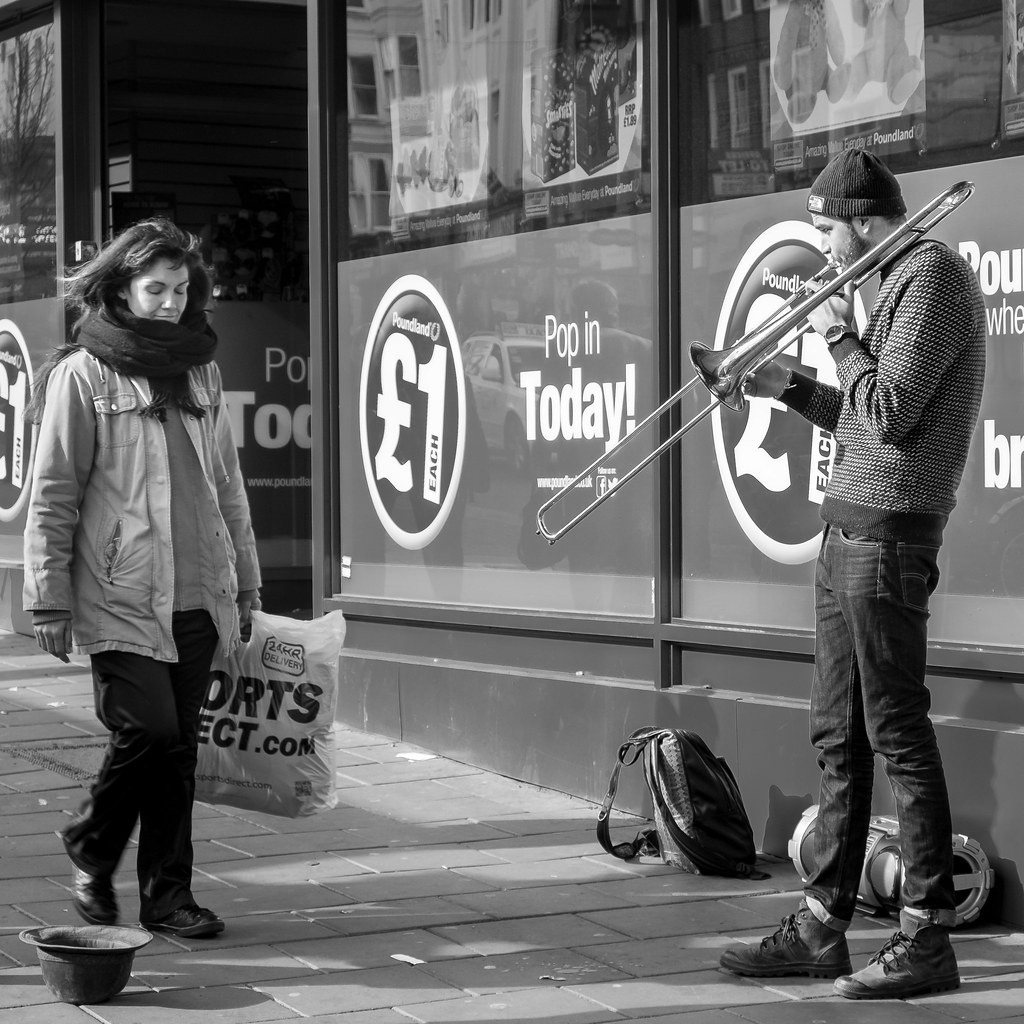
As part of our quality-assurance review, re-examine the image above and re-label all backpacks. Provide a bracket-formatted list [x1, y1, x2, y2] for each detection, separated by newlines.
[595, 725, 773, 881]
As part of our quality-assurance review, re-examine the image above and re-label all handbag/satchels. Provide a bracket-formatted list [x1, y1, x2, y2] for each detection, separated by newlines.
[193, 609, 348, 819]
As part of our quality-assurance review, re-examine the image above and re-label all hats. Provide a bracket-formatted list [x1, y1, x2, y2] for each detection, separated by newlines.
[805, 147, 909, 218]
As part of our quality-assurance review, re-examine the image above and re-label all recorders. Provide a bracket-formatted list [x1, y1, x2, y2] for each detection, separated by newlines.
[787, 805, 994, 925]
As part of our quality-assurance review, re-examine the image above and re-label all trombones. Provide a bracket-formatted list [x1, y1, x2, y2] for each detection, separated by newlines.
[534, 181, 974, 546]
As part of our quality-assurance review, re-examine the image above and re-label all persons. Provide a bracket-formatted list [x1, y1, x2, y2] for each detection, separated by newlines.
[722, 146, 984, 997]
[23, 218, 264, 936]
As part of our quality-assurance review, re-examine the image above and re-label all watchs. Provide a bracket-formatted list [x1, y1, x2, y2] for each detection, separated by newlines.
[824, 324, 853, 344]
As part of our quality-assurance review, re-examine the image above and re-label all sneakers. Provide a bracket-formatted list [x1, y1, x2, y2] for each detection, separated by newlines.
[833, 909, 960, 999]
[719, 899, 854, 980]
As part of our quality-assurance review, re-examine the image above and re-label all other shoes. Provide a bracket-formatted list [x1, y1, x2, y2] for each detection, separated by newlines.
[71, 862, 119, 925]
[140, 900, 225, 938]
